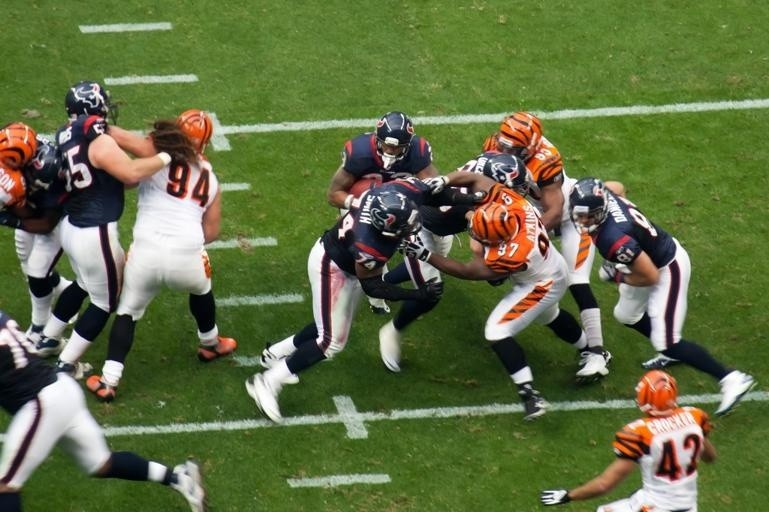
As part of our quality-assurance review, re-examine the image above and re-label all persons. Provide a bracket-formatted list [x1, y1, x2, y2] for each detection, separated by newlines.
[480, 112, 611, 383]
[0, 315, 211, 512]
[0, 121, 68, 341]
[55, 81, 182, 383]
[540, 367, 716, 512]
[567, 178, 760, 417]
[71, 109, 238, 399]
[371, 147, 546, 372]
[401, 169, 608, 425]
[243, 184, 487, 423]
[322, 109, 452, 209]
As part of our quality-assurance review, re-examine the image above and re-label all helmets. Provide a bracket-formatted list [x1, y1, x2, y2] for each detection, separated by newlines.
[636, 370, 677, 418]
[485, 151, 531, 199]
[570, 177, 608, 234]
[177, 109, 212, 153]
[0, 122, 36, 166]
[469, 203, 519, 246]
[66, 80, 109, 121]
[370, 190, 422, 239]
[497, 112, 544, 161]
[1, 161, 25, 206]
[376, 112, 414, 170]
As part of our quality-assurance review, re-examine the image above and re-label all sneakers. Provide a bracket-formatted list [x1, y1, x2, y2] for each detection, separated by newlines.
[524, 393, 552, 421]
[246, 372, 282, 425]
[259, 343, 300, 385]
[642, 353, 680, 370]
[36, 335, 68, 357]
[380, 320, 401, 374]
[713, 372, 757, 416]
[582, 343, 613, 369]
[26, 324, 43, 343]
[85, 376, 116, 401]
[61, 280, 80, 326]
[174, 461, 206, 511]
[573, 353, 610, 389]
[198, 336, 235, 362]
[57, 358, 93, 380]
[367, 296, 391, 315]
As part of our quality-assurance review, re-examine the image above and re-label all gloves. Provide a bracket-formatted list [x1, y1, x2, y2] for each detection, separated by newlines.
[600, 263, 624, 282]
[23, 217, 44, 232]
[423, 276, 444, 300]
[424, 175, 447, 195]
[159, 149, 186, 166]
[542, 488, 568, 506]
[75, 113, 108, 137]
[349, 196, 358, 210]
[399, 234, 432, 263]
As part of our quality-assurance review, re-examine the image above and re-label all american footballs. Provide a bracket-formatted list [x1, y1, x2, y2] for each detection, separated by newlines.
[349, 180, 382, 200]
